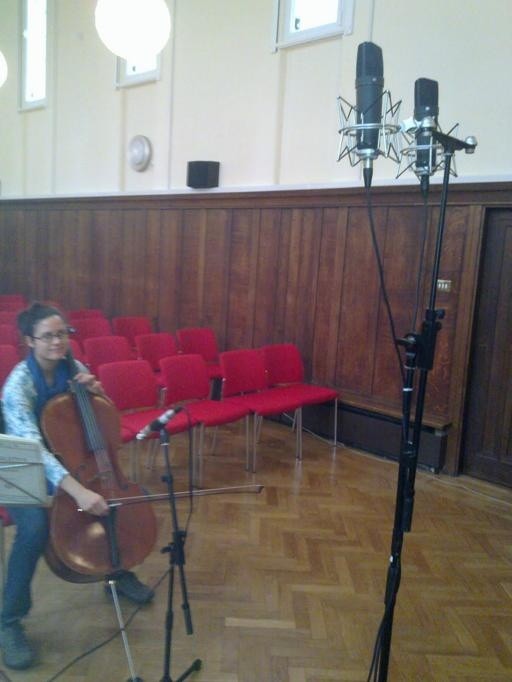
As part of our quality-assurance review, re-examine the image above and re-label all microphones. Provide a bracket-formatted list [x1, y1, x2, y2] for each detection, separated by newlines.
[416, 76, 438, 174]
[357, 41, 384, 166]
[136, 405, 179, 441]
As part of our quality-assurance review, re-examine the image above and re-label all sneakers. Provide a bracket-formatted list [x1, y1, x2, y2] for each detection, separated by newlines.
[1, 621, 33, 670]
[106, 571, 155, 604]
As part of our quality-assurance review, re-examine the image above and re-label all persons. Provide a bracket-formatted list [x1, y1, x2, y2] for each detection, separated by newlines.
[0, 301, 156, 673]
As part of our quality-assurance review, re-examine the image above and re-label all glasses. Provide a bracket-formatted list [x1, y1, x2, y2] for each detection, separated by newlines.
[32, 324, 77, 342]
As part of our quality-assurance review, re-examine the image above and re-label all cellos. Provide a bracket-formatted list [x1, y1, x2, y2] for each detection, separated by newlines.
[41, 324, 157, 583]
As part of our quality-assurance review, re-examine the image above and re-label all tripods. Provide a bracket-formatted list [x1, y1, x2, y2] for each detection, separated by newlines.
[125, 433, 202, 682]
